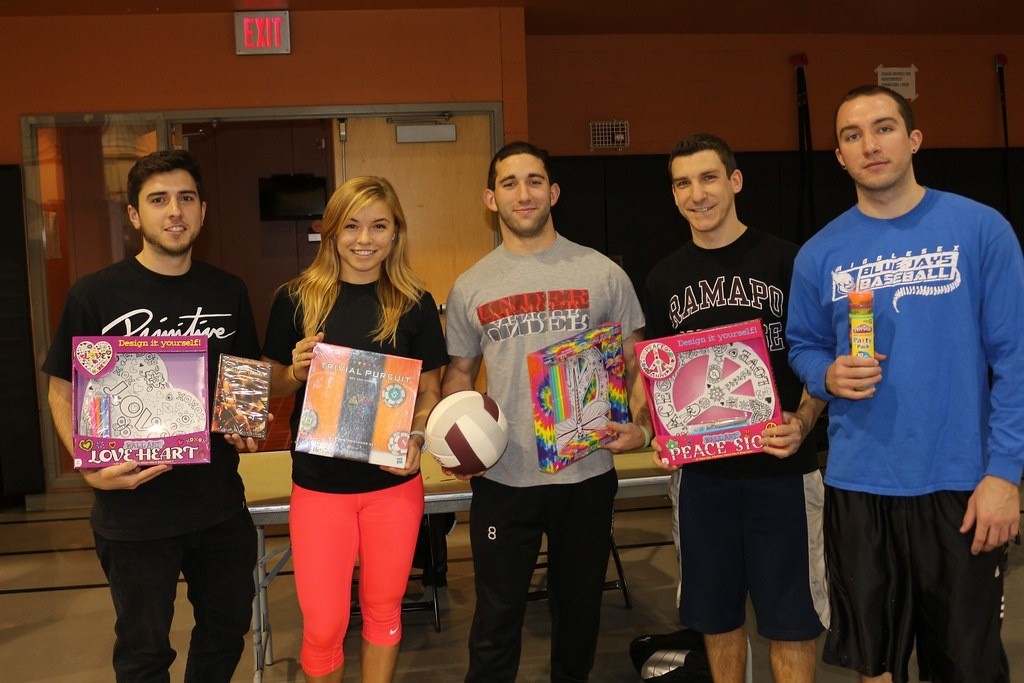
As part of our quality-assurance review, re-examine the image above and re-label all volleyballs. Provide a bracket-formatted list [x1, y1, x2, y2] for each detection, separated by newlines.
[423, 390, 508, 474]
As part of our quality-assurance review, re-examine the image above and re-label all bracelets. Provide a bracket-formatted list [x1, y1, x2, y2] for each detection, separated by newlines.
[639, 423, 652, 449]
[409, 431, 426, 442]
[291, 364, 305, 384]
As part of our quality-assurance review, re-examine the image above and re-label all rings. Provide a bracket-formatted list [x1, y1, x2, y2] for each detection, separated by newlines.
[294, 354, 300, 362]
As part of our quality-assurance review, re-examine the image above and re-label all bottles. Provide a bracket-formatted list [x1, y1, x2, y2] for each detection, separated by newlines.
[847, 292, 875, 393]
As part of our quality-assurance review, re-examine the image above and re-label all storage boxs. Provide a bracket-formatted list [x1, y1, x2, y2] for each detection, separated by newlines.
[526, 321, 631, 473]
[294, 341, 423, 472]
[633, 315, 790, 469]
[68, 330, 213, 471]
[209, 352, 274, 443]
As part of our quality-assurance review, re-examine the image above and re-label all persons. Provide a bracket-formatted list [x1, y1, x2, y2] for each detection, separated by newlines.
[782, 85, 1024, 683]
[638, 133, 828, 683]
[443, 140, 648, 683]
[261, 175, 450, 683]
[39, 150, 273, 683]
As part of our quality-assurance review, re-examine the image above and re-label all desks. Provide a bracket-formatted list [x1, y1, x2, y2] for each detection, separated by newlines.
[243, 473, 684, 683]
